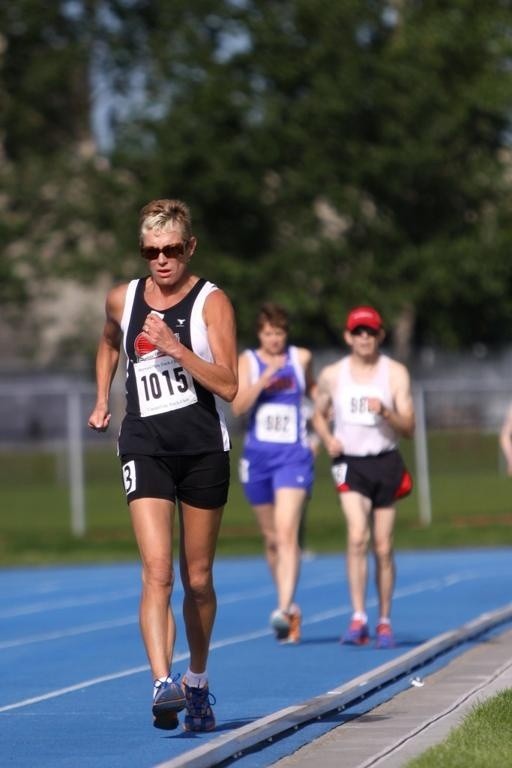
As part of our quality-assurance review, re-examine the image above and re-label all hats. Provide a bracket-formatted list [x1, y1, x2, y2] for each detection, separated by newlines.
[347, 307, 382, 331]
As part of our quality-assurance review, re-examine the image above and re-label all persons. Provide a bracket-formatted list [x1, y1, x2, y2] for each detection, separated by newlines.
[86, 199, 239, 735]
[499, 406, 512, 476]
[311, 305, 417, 649]
[231, 304, 321, 646]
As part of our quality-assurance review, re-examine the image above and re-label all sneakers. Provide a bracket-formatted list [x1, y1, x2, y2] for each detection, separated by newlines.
[342, 621, 392, 648]
[153, 673, 216, 731]
[272, 604, 301, 643]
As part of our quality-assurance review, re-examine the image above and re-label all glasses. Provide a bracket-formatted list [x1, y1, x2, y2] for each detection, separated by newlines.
[140, 242, 184, 258]
[352, 327, 377, 336]
